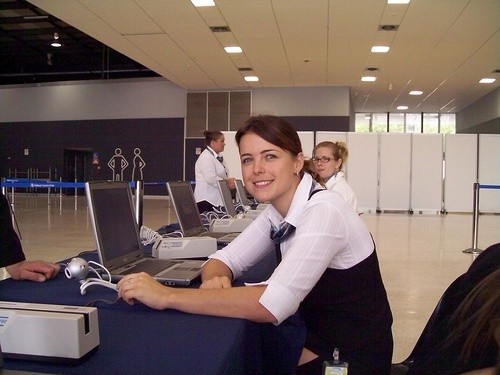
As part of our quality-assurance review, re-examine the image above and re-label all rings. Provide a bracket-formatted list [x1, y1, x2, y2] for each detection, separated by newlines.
[126, 278, 132, 285]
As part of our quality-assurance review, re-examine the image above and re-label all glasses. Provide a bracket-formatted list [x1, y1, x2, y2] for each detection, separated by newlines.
[310, 156, 338, 164]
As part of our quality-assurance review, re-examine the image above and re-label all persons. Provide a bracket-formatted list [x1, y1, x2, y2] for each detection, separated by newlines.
[312, 141, 359, 215]
[193, 130, 235, 216]
[116, 113, 393, 375]
[0, 192, 61, 283]
[445, 271, 500, 375]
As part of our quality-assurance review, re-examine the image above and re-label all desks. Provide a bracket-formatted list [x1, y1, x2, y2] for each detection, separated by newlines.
[0, 211, 308, 375]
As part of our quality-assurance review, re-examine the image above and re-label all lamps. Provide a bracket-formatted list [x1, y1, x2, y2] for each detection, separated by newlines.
[51, 31, 61, 48]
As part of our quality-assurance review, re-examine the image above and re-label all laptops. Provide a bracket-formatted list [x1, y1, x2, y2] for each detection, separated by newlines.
[85, 180, 207, 287]
[166, 181, 241, 245]
[217, 180, 237, 219]
[235, 180, 249, 207]
